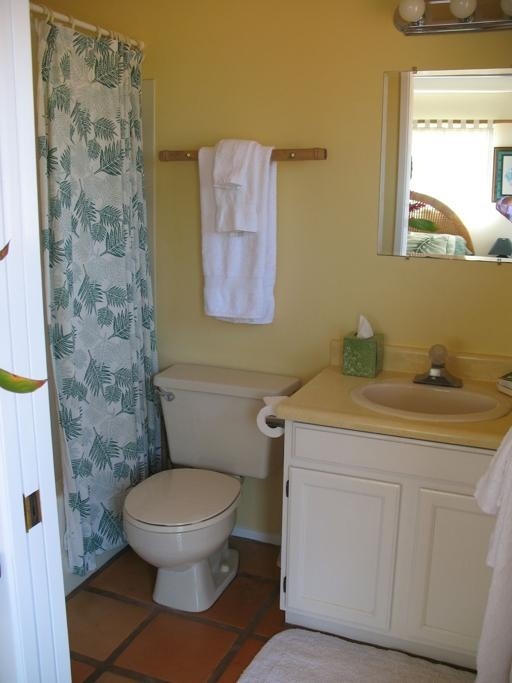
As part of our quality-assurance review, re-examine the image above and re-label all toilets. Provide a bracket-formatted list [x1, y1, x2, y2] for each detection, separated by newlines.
[122, 362, 300, 613]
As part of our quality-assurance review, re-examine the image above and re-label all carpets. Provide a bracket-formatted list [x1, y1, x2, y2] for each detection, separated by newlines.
[233, 630, 476, 681]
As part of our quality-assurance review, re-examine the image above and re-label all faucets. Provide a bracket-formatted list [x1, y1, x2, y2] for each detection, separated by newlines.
[413, 345, 462, 387]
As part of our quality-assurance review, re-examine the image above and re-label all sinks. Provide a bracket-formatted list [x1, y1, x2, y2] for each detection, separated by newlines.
[350, 380, 512, 423]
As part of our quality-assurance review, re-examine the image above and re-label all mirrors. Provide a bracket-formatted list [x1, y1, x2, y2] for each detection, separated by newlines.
[374, 67, 512, 263]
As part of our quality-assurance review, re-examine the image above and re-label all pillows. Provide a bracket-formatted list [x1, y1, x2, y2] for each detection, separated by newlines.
[405, 231, 474, 257]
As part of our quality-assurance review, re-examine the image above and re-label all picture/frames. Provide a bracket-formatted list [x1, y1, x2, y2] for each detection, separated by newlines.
[492, 145, 512, 202]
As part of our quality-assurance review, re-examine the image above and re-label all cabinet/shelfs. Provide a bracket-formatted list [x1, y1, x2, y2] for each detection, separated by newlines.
[270, 419, 497, 672]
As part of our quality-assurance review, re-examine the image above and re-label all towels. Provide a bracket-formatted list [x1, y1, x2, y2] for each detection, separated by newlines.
[468, 428, 512, 680]
[196, 137, 279, 327]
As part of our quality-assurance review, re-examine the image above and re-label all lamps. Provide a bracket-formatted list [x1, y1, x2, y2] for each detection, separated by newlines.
[391, 0, 511, 36]
[488, 237, 512, 259]
